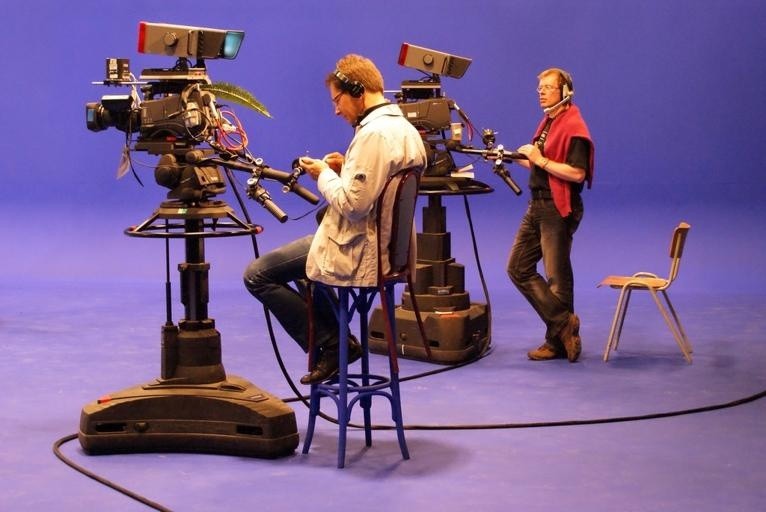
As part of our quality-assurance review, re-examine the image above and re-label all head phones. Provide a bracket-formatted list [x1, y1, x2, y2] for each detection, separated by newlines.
[561, 82, 576, 100]
[332, 67, 365, 98]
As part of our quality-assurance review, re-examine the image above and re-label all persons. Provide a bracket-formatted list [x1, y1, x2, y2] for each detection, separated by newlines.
[242, 53, 428, 385]
[483, 68, 596, 363]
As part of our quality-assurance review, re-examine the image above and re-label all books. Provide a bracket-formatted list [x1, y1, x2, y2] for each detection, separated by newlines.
[450, 163, 475, 178]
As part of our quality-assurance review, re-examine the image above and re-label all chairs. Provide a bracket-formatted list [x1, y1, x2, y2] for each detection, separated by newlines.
[302, 166, 432, 371]
[596, 222, 695, 363]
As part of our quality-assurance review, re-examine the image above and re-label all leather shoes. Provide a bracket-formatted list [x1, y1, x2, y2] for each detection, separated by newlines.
[299, 334, 363, 386]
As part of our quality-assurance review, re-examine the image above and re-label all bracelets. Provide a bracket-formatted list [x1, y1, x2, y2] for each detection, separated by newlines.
[538, 157, 550, 169]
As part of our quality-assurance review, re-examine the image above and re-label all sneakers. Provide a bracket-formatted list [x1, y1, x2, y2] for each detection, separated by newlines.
[527, 342, 562, 360]
[558, 312, 582, 362]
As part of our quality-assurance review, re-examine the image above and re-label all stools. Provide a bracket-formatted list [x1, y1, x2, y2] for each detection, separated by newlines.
[301, 280, 409, 468]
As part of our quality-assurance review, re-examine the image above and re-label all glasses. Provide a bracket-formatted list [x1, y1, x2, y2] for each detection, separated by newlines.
[535, 84, 562, 92]
[329, 91, 349, 107]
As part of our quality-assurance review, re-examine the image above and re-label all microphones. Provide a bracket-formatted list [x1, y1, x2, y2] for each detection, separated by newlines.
[542, 96, 573, 115]
